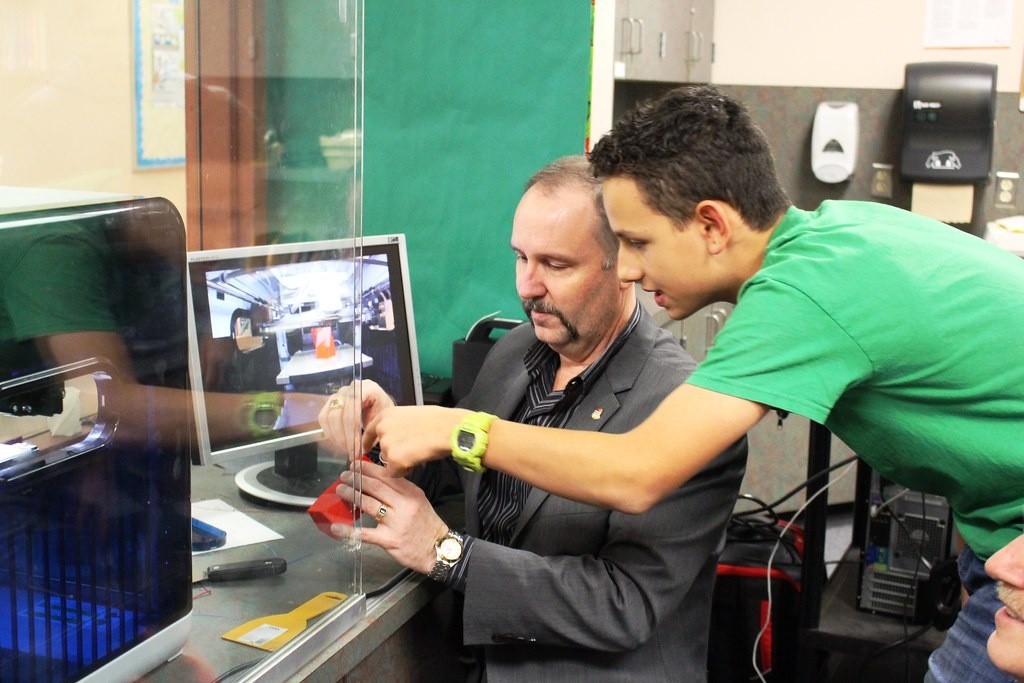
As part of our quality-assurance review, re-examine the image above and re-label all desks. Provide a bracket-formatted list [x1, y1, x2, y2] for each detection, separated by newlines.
[275, 348, 373, 396]
[126, 443, 444, 683]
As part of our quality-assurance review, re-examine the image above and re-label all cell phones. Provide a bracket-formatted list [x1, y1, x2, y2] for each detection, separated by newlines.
[207, 558, 287, 582]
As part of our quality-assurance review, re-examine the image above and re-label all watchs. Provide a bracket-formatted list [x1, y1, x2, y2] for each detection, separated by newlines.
[450, 412, 500, 474]
[250, 391, 288, 441]
[425, 528, 466, 582]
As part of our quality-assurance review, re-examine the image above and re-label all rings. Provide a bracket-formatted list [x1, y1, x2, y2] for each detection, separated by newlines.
[374, 504, 388, 522]
[378, 451, 388, 465]
[325, 398, 344, 411]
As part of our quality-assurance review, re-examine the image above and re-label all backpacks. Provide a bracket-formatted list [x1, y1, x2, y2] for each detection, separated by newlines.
[706, 516, 827, 683]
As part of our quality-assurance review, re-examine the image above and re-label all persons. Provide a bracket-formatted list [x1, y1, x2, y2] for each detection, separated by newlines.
[302, 154, 750, 683]
[0, 208, 331, 450]
[984, 515, 1024, 680]
[362, 83, 1024, 683]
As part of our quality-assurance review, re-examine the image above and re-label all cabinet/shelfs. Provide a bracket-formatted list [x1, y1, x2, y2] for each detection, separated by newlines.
[612, 0, 723, 81]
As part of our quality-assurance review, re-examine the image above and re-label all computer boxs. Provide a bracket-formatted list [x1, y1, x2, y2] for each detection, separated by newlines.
[854, 451, 961, 625]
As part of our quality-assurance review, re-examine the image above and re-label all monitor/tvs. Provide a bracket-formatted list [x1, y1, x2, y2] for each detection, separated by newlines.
[186, 233, 425, 510]
